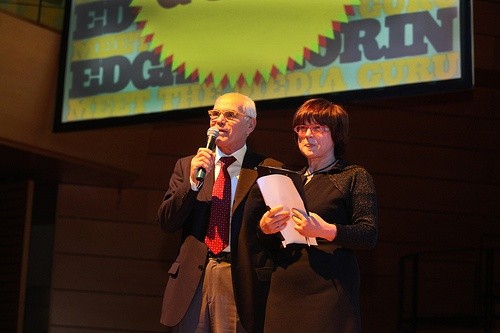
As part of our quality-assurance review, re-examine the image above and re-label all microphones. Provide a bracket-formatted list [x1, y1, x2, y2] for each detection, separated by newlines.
[196, 127, 219, 182]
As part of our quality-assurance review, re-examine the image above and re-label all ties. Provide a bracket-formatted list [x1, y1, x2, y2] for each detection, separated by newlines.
[203, 154, 239, 255]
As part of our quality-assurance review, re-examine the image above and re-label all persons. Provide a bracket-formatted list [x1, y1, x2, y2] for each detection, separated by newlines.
[259, 96, 378, 332]
[155, 93, 285, 333]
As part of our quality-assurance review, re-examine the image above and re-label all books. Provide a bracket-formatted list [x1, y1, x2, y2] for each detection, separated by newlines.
[256, 164, 319, 249]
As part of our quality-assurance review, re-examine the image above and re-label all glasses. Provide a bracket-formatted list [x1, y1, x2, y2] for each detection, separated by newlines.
[293, 124, 334, 136]
[208, 109, 250, 121]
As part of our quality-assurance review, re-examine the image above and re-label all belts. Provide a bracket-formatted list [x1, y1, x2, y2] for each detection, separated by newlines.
[206, 250, 232, 265]
